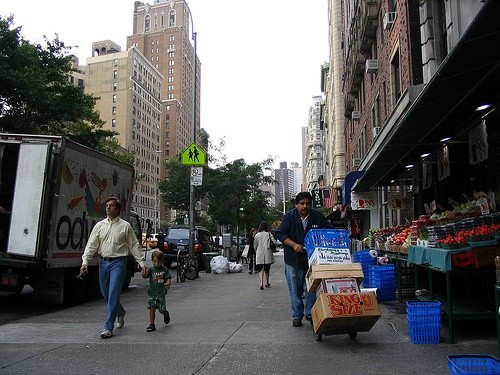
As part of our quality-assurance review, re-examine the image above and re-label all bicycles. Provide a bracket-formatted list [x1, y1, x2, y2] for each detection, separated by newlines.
[178, 254, 200, 282]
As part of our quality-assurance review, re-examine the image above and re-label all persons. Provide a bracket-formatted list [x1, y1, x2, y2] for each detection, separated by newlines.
[142, 251, 171, 332]
[246, 227, 258, 274]
[0, 206, 11, 215]
[274, 192, 329, 327]
[79, 197, 148, 338]
[253, 221, 279, 290]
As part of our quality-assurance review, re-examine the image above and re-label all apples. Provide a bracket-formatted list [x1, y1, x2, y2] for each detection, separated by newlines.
[369, 227, 394, 236]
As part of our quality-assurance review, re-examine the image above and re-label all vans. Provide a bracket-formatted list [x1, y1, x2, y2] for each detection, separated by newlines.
[211, 235, 222, 245]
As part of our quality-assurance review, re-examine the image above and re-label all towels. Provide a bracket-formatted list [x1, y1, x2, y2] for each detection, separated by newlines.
[425, 247, 451, 271]
[407, 244, 426, 265]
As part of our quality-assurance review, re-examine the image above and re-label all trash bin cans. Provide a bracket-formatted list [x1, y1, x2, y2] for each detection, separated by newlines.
[203, 252, 221, 273]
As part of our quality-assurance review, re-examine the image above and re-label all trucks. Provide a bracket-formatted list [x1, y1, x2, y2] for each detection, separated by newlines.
[0, 132, 153, 307]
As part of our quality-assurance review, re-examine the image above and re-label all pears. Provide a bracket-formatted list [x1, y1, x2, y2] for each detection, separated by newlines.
[434, 203, 465, 224]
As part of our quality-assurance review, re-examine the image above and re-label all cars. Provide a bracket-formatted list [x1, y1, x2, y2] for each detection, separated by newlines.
[233, 237, 246, 245]
[144, 234, 159, 249]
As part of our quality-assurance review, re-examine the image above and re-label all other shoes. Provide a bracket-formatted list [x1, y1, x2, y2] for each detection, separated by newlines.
[248, 270, 252, 274]
[260, 286, 264, 290]
[164, 310, 170, 324]
[255, 271, 257, 273]
[100, 330, 113, 338]
[116, 308, 126, 328]
[293, 319, 303, 327]
[146, 323, 156, 332]
[265, 284, 270, 288]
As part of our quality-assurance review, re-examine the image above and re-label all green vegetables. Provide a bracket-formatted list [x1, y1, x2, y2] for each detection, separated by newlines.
[418, 227, 428, 241]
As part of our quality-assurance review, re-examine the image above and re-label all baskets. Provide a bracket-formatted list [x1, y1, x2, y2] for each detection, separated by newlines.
[447, 354, 500, 375]
[304, 228, 349, 259]
[406, 300, 443, 345]
[353, 250, 396, 303]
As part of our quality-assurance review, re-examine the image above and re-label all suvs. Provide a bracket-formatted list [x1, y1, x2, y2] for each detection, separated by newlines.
[162, 225, 215, 269]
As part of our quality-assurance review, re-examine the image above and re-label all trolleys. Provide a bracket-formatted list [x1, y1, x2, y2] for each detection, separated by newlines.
[301, 246, 359, 343]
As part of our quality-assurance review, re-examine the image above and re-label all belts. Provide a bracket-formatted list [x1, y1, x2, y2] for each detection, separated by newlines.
[99, 255, 119, 261]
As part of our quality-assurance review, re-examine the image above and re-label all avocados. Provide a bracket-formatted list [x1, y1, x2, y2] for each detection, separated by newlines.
[379, 230, 399, 243]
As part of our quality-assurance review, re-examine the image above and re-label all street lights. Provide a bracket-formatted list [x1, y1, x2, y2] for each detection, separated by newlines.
[265, 167, 285, 216]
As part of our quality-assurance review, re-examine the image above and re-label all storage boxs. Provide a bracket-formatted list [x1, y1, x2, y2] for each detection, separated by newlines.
[304, 227, 500, 344]
[446, 353, 500, 375]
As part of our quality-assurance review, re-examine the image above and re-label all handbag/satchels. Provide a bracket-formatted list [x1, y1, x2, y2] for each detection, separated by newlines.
[241, 245, 249, 258]
[269, 232, 276, 253]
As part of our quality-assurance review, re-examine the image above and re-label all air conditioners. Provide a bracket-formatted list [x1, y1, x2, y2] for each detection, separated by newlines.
[353, 158, 362, 167]
[352, 111, 360, 121]
[382, 12, 397, 30]
[365, 58, 379, 73]
[373, 127, 381, 137]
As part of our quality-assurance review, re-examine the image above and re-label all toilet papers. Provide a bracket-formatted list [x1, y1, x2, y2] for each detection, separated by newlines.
[378, 257, 387, 265]
[369, 250, 377, 258]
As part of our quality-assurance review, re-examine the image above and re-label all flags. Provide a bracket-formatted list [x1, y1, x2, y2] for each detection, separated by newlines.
[323, 189, 337, 208]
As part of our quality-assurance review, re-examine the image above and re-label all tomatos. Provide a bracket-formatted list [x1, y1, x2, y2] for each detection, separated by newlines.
[395, 227, 412, 243]
